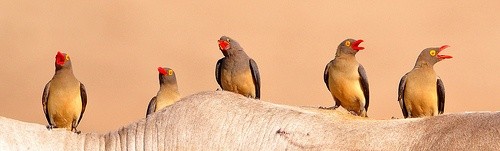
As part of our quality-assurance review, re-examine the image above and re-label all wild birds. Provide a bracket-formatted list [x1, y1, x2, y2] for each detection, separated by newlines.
[42, 51, 88, 134]
[318, 39, 370, 116]
[397, 45, 453, 118]
[146, 67, 181, 117]
[215, 36, 261, 99]
